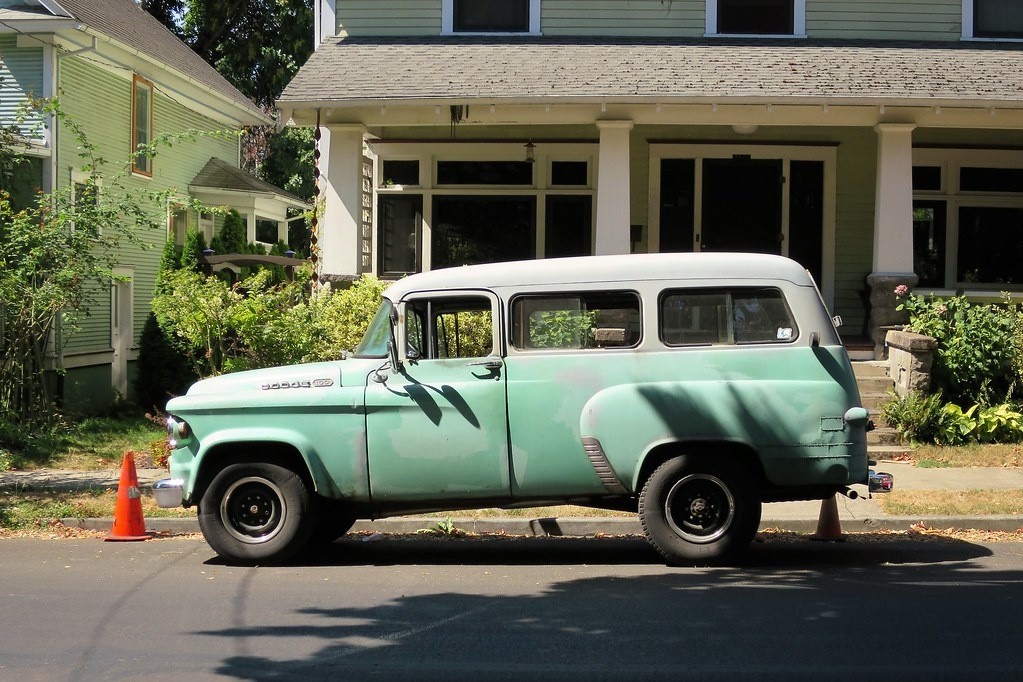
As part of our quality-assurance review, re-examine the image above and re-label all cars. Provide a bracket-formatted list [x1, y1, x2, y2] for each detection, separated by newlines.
[154, 252, 875, 563]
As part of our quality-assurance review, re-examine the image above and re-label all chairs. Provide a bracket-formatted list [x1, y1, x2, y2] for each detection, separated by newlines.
[593, 305, 631, 350]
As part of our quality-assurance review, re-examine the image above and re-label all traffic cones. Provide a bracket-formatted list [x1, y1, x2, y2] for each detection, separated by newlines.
[103, 451, 154, 542]
